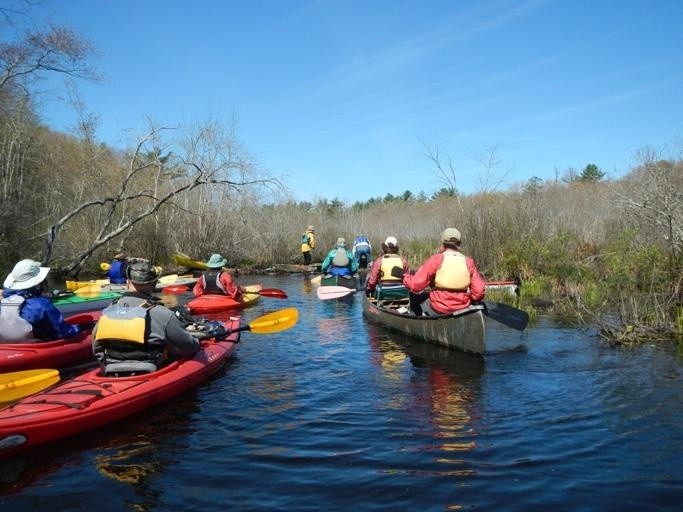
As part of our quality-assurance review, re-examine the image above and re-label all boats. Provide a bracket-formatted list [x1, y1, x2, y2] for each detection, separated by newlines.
[319, 272, 355, 303]
[0, 312, 244, 452]
[362, 272, 488, 357]
[321, 292, 355, 320]
[363, 314, 488, 432]
[1, 247, 263, 370]
[356, 268, 370, 290]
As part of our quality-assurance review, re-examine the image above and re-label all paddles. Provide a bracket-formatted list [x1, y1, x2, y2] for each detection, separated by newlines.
[477, 300, 529, 331]
[199, 308, 300, 341]
[238, 289, 288, 298]
[317, 285, 365, 300]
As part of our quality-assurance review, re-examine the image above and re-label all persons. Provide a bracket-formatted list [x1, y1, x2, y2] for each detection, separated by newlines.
[402, 228, 485, 316]
[301, 226, 315, 265]
[353, 236, 373, 270]
[0, 258, 95, 344]
[192, 254, 246, 299]
[321, 238, 359, 280]
[107, 254, 128, 284]
[366, 236, 410, 297]
[92, 263, 200, 377]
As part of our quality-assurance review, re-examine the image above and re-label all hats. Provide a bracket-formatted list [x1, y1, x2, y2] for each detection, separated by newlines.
[125, 262, 162, 285]
[2, 257, 51, 291]
[308, 225, 316, 231]
[384, 236, 398, 249]
[205, 253, 228, 269]
[440, 227, 463, 243]
